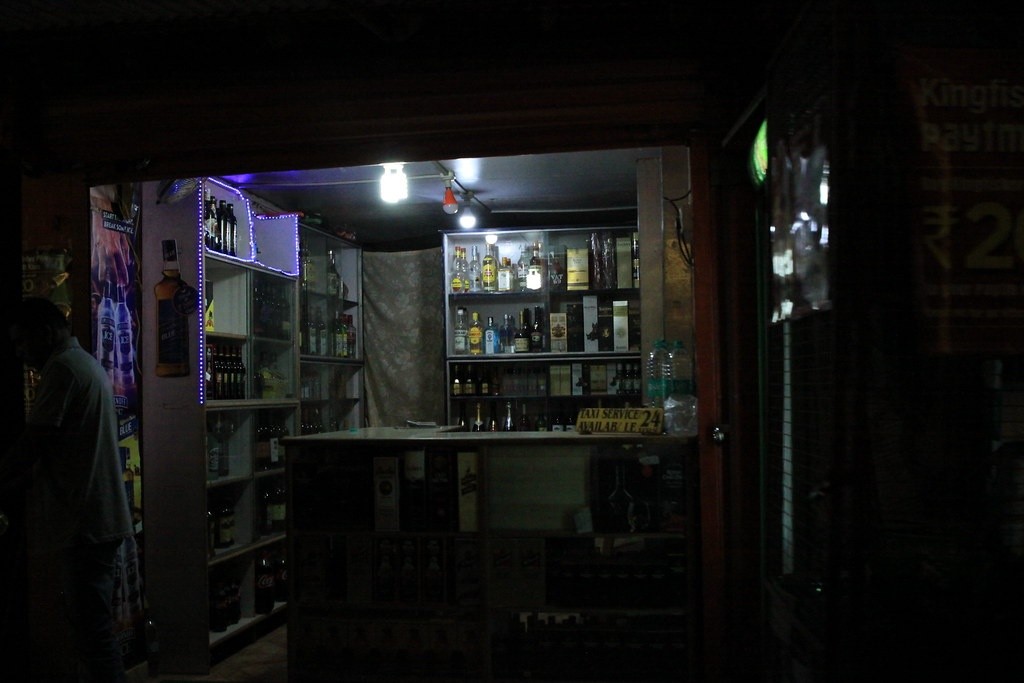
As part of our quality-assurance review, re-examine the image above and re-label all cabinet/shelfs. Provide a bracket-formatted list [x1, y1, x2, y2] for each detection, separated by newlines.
[276, 428, 700, 683]
[138, 175, 300, 674]
[435, 222, 643, 355]
[301, 358, 365, 430]
[295, 221, 366, 363]
[444, 355, 642, 426]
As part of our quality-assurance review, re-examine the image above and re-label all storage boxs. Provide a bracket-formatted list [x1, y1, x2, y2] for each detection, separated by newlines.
[299, 230, 688, 683]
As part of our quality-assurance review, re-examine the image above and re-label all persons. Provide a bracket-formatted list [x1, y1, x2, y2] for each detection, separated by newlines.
[1, 296, 136, 683]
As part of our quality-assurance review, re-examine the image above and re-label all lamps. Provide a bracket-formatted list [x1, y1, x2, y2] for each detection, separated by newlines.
[440, 170, 459, 214]
[458, 191, 477, 229]
[376, 162, 410, 205]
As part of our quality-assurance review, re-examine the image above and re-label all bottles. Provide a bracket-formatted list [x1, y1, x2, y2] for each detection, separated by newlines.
[112, 448, 149, 670]
[96, 281, 134, 391]
[607, 466, 633, 533]
[617, 364, 640, 394]
[454, 305, 544, 355]
[460, 400, 577, 432]
[300, 239, 356, 358]
[154, 240, 191, 378]
[206, 342, 284, 400]
[301, 366, 349, 435]
[207, 411, 290, 632]
[642, 338, 698, 437]
[586, 232, 616, 289]
[50, 248, 72, 329]
[254, 280, 291, 340]
[205, 196, 237, 256]
[449, 364, 546, 396]
[452, 242, 544, 292]
